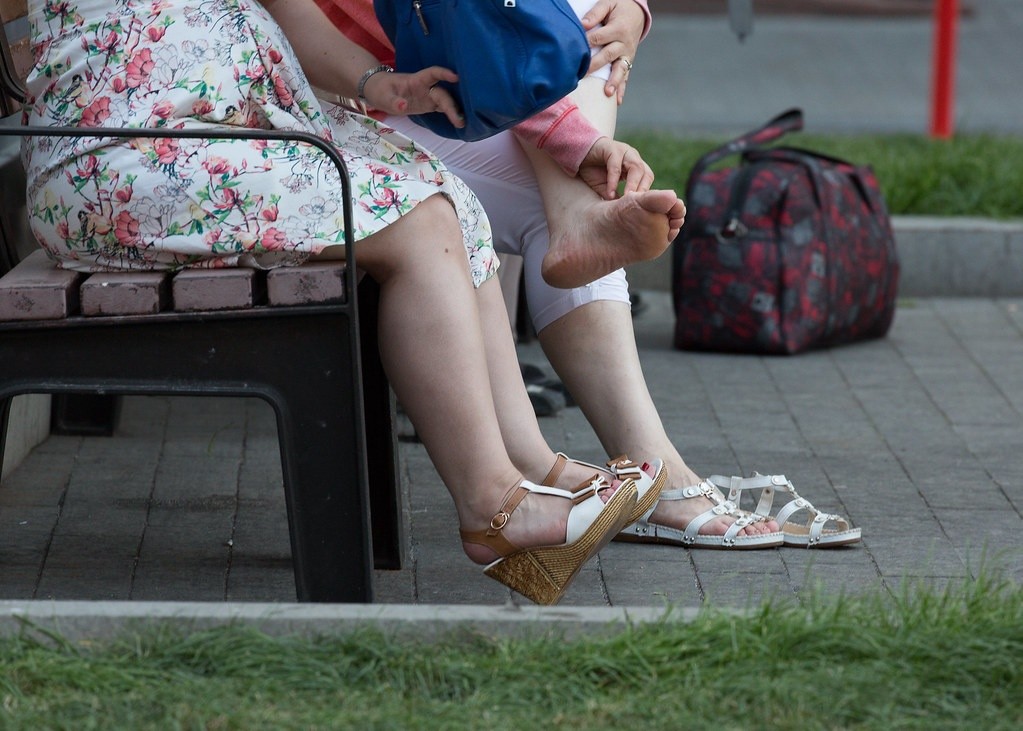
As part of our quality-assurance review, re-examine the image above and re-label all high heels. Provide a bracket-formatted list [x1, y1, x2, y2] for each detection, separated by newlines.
[541, 452, 667, 530]
[461, 481, 638, 606]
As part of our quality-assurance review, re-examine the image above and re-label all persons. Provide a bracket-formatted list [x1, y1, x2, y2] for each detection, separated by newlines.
[22, 0, 785, 605]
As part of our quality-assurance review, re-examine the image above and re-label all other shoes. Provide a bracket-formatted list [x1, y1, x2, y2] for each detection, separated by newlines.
[526, 380, 574, 414]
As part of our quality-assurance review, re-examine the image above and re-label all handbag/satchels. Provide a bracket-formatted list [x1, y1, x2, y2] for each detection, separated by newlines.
[672, 109, 901, 355]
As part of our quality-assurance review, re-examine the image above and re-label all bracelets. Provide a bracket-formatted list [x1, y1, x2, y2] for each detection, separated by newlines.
[357, 65, 393, 98]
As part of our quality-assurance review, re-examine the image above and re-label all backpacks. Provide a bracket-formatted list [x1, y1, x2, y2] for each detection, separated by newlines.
[372, 0, 590, 143]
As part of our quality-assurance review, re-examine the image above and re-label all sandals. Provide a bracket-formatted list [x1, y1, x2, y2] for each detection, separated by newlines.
[620, 479, 784, 546]
[711, 473, 864, 545]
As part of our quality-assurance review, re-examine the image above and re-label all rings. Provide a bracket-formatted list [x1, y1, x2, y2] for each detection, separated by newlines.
[429, 86, 435, 93]
[617, 58, 632, 70]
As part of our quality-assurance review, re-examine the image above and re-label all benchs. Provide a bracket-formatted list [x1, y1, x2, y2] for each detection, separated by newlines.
[0, 18, 406, 604]
